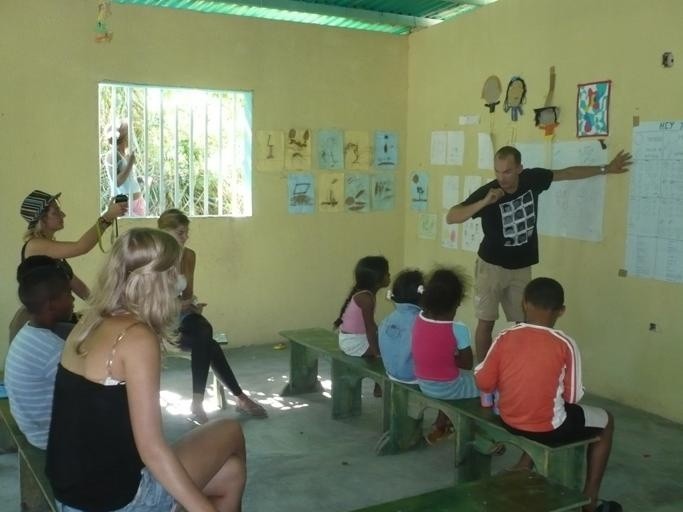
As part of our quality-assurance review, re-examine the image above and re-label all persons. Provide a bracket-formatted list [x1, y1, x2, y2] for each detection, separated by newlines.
[473, 277, 624, 511]
[42, 227, 247, 510]
[410, 264, 480, 447]
[376, 268, 425, 385]
[3, 268, 74, 450]
[104, 119, 145, 217]
[445, 146, 633, 364]
[18, 190, 129, 316]
[156, 208, 267, 424]
[7, 255, 72, 345]
[332, 257, 390, 398]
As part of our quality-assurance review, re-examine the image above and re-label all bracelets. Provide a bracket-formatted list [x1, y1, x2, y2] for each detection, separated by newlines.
[99, 215, 111, 226]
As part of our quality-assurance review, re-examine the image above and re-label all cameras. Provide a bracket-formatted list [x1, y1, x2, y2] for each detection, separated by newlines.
[116, 195, 129, 216]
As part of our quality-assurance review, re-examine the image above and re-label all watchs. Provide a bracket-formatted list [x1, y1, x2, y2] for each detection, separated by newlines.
[600, 164, 606, 175]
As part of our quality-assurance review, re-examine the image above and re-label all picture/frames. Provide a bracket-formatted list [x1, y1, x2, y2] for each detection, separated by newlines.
[575, 79, 612, 138]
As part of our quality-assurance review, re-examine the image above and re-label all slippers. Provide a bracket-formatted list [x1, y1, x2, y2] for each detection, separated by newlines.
[595, 498, 622, 512]
[235, 405, 269, 419]
[187, 414, 207, 426]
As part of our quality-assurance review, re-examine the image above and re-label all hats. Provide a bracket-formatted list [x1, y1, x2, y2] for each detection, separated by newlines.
[20, 190, 62, 229]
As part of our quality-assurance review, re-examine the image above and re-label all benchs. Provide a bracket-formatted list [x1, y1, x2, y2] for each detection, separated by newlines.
[0, 394, 58, 511]
[345, 468, 592, 511]
[207, 332, 232, 410]
[278, 326, 600, 492]
